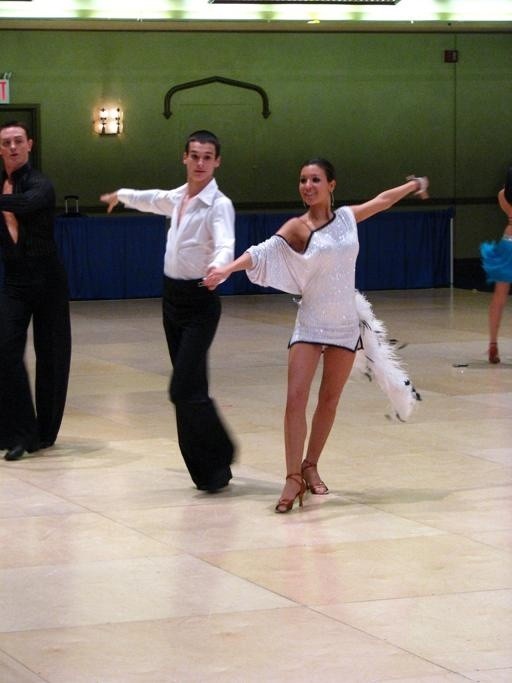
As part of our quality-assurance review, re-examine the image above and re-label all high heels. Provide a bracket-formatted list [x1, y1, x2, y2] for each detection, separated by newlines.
[5, 440, 51, 460]
[489, 342, 500, 364]
[302, 459, 329, 494]
[276, 473, 308, 513]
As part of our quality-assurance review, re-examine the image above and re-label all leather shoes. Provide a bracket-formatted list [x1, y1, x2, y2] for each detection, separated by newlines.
[197, 465, 232, 489]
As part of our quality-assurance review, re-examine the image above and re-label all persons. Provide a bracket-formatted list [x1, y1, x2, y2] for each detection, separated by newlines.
[100, 130, 235, 492]
[203, 159, 430, 514]
[1, 120, 71, 462]
[479, 187, 512, 363]
